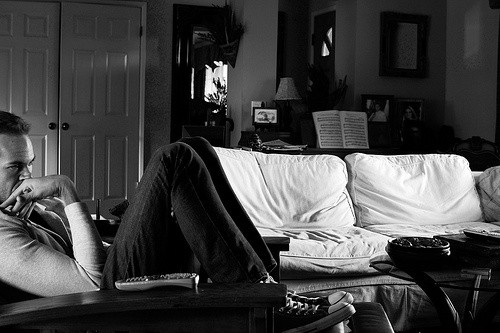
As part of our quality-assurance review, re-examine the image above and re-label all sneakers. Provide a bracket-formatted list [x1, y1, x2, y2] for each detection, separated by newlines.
[288, 288, 354, 306]
[276, 297, 356, 333]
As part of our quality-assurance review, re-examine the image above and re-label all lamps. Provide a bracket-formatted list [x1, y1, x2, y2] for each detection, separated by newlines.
[271, 77, 303, 132]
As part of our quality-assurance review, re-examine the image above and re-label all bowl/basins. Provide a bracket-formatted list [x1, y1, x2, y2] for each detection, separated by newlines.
[385, 235, 452, 269]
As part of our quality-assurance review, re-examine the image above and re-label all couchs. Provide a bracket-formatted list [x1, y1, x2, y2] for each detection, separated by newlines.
[212, 147, 500, 333]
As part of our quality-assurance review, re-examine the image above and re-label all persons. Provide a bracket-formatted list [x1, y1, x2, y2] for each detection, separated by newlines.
[0, 111, 355, 333]
[402, 107, 418, 122]
[368, 99, 390, 122]
[257, 112, 275, 122]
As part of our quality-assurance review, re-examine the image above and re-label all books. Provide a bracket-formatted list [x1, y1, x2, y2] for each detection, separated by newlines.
[262, 139, 308, 151]
[313, 112, 369, 150]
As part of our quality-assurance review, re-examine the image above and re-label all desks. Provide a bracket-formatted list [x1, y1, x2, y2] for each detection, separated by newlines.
[238, 131, 292, 148]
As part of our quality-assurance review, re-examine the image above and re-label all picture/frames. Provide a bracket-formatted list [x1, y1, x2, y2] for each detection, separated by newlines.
[380, 11, 429, 79]
[363, 95, 425, 122]
[252, 107, 279, 125]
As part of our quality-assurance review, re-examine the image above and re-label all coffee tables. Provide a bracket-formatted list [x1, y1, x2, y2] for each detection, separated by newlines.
[370, 254, 500, 333]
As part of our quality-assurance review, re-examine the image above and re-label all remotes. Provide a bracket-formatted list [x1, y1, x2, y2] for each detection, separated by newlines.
[463, 228, 500, 243]
[114, 272, 200, 290]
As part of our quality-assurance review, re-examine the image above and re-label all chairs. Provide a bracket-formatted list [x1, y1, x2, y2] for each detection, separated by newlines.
[0, 220, 290, 333]
